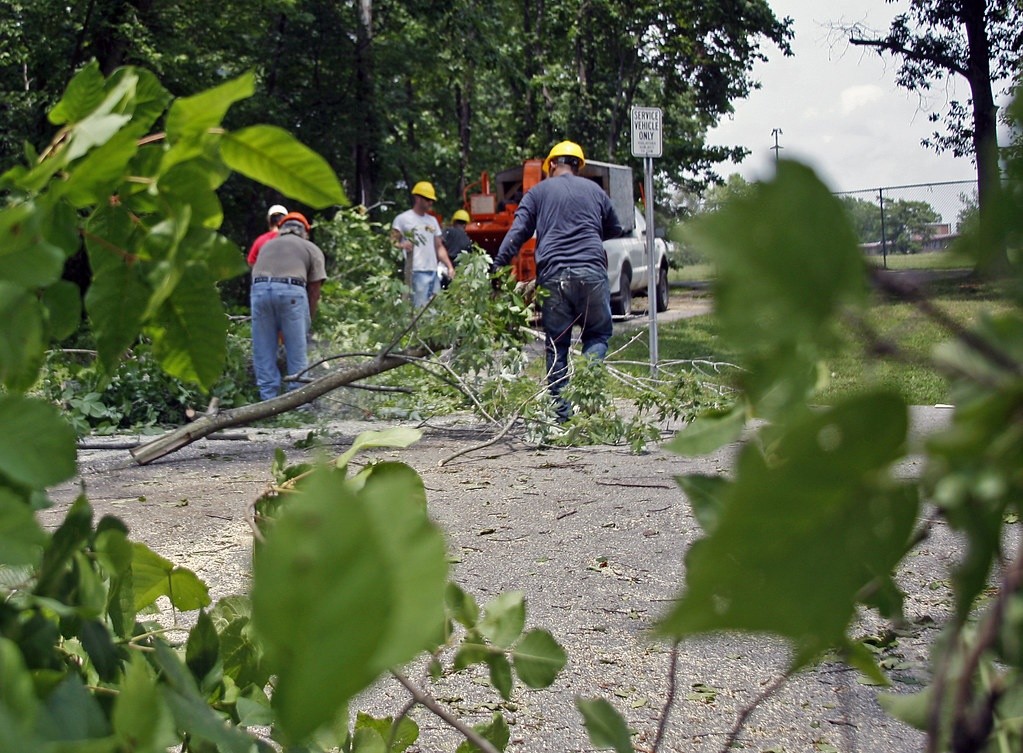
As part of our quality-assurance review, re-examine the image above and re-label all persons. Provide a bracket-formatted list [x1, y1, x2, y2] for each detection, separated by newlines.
[389, 180, 472, 312]
[247, 204, 328, 402]
[489, 139, 624, 423]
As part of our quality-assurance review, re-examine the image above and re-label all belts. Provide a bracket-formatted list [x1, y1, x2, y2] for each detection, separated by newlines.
[255, 278, 304, 287]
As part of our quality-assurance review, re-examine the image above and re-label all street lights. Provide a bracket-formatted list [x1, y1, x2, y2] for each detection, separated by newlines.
[770, 127, 783, 160]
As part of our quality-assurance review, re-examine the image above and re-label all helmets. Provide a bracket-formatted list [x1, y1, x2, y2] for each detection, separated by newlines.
[542, 141, 585, 173]
[451, 210, 470, 225]
[411, 182, 437, 202]
[277, 212, 310, 234]
[267, 205, 288, 223]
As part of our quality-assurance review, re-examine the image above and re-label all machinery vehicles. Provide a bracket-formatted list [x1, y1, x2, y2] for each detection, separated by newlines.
[458, 159, 646, 287]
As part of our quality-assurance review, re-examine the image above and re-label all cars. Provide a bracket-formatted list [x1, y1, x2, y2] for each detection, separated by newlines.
[599, 208, 674, 319]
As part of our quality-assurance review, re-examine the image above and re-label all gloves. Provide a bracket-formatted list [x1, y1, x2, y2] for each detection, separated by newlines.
[491, 266, 501, 292]
